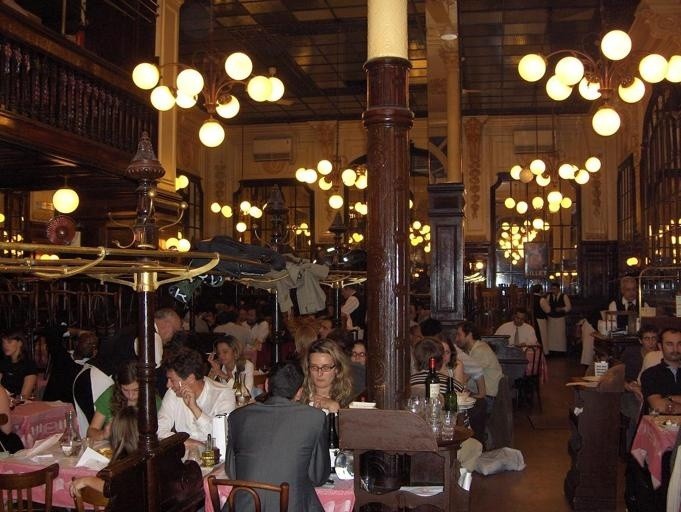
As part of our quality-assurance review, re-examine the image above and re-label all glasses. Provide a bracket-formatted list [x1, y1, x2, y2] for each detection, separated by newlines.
[308, 365, 337, 373]
[349, 351, 366, 357]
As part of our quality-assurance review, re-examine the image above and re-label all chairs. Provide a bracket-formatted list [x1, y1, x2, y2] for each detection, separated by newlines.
[0, 291, 680, 511]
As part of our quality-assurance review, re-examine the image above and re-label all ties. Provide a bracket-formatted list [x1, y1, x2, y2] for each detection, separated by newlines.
[515, 327, 520, 345]
[627, 301, 630, 310]
[555, 294, 557, 301]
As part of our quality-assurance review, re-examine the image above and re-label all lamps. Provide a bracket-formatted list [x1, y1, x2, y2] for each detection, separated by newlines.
[51, 9, 486, 285]
[497, 28, 680, 279]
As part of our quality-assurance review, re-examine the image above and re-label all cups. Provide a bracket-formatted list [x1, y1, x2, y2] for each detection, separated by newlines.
[9, 390, 36, 411]
[665, 396, 676, 423]
[400, 393, 457, 442]
[649, 407, 660, 421]
[81, 436, 96, 453]
[188, 447, 201, 464]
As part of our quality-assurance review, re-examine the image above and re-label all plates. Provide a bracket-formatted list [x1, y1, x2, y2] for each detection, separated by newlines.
[349, 401, 378, 410]
[662, 424, 681, 430]
[583, 375, 605, 382]
[97, 447, 114, 460]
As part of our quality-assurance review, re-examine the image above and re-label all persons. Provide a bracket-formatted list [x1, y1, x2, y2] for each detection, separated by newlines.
[1, 308, 183, 510]
[563, 277, 681, 511]
[495, 283, 572, 405]
[407, 302, 504, 451]
[155, 284, 366, 511]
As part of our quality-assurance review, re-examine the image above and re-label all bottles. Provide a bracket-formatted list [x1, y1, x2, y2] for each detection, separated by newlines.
[426, 357, 441, 403]
[502, 283, 508, 296]
[204, 433, 215, 466]
[326, 411, 340, 473]
[459, 467, 474, 490]
[232, 370, 251, 407]
[59, 410, 82, 456]
[445, 375, 458, 413]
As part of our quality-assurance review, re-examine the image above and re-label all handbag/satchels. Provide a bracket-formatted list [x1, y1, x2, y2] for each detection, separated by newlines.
[476, 447, 525, 476]
